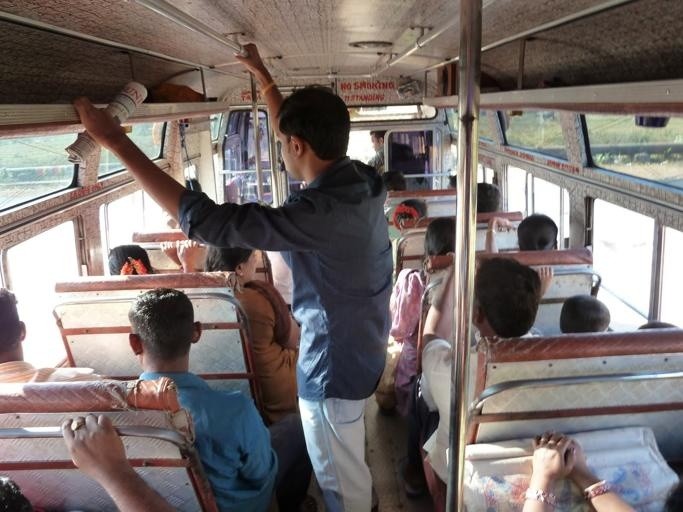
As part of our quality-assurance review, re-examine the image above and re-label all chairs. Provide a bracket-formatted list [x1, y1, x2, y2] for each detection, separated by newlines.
[384, 189, 523, 284]
[416, 247, 593, 374]
[0, 376, 218, 512]
[52, 271, 297, 427]
[418, 328, 683, 512]
[132, 230, 273, 286]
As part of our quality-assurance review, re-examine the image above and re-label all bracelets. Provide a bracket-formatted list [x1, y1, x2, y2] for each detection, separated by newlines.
[583, 480, 610, 498]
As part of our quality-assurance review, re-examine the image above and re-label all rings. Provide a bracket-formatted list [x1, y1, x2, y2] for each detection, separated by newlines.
[69, 420, 85, 430]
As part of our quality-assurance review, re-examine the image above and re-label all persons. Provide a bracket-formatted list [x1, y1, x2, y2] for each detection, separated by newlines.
[73, 41, 392, 512]
[474, 183, 499, 213]
[0, 289, 104, 385]
[1, 416, 183, 512]
[389, 198, 428, 275]
[446, 174, 457, 189]
[202, 240, 302, 424]
[126, 289, 316, 512]
[108, 239, 199, 275]
[374, 218, 456, 417]
[405, 257, 554, 496]
[366, 132, 392, 169]
[381, 172, 406, 209]
[486, 212, 559, 255]
[560, 294, 610, 333]
[523, 431, 683, 512]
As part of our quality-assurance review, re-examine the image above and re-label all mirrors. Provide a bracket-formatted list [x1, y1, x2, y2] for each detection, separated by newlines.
[347, 101, 438, 122]
